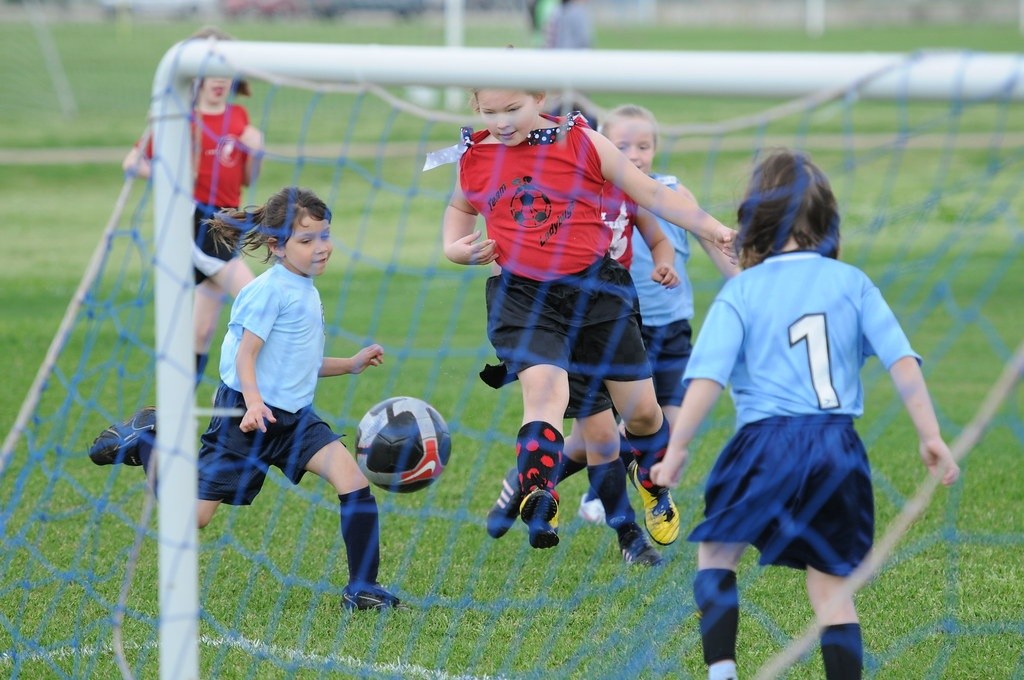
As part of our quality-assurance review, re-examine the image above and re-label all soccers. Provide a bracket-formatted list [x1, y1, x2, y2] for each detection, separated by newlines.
[354, 395, 453, 494]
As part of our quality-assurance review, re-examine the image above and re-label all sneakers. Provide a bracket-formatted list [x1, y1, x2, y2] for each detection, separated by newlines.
[520, 488, 559, 551]
[343, 584, 405, 611]
[90, 406, 155, 467]
[628, 460, 681, 545]
[577, 492, 606, 523]
[618, 522, 662, 568]
[486, 468, 524, 540]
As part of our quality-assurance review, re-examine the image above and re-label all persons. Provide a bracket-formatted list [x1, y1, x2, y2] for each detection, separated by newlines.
[442, 88, 742, 549]
[123, 27, 264, 397]
[531, 0, 596, 129]
[650, 151, 961, 680]
[579, 105, 742, 525]
[486, 94, 681, 566]
[89, 187, 398, 610]
